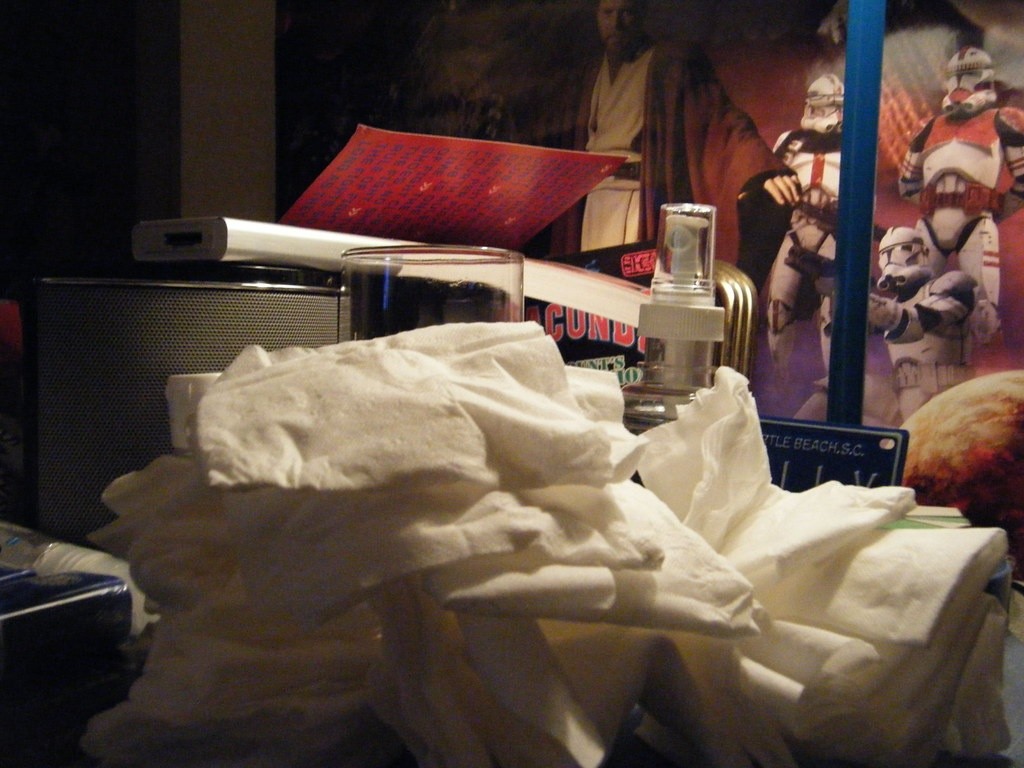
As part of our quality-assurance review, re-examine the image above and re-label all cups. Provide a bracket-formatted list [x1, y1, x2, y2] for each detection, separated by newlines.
[343, 246, 522, 339]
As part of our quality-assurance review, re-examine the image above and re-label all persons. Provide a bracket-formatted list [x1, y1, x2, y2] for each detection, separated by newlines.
[552, 2, 1020, 516]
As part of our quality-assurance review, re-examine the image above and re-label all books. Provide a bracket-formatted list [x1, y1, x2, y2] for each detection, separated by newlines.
[123, 217, 650, 323]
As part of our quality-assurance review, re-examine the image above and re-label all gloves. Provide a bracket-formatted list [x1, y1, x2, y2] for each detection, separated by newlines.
[867, 293, 904, 333]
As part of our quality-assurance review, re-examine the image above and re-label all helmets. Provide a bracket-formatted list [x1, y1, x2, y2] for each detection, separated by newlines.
[877, 225, 933, 296]
[940, 44, 997, 116]
[800, 73, 844, 134]
